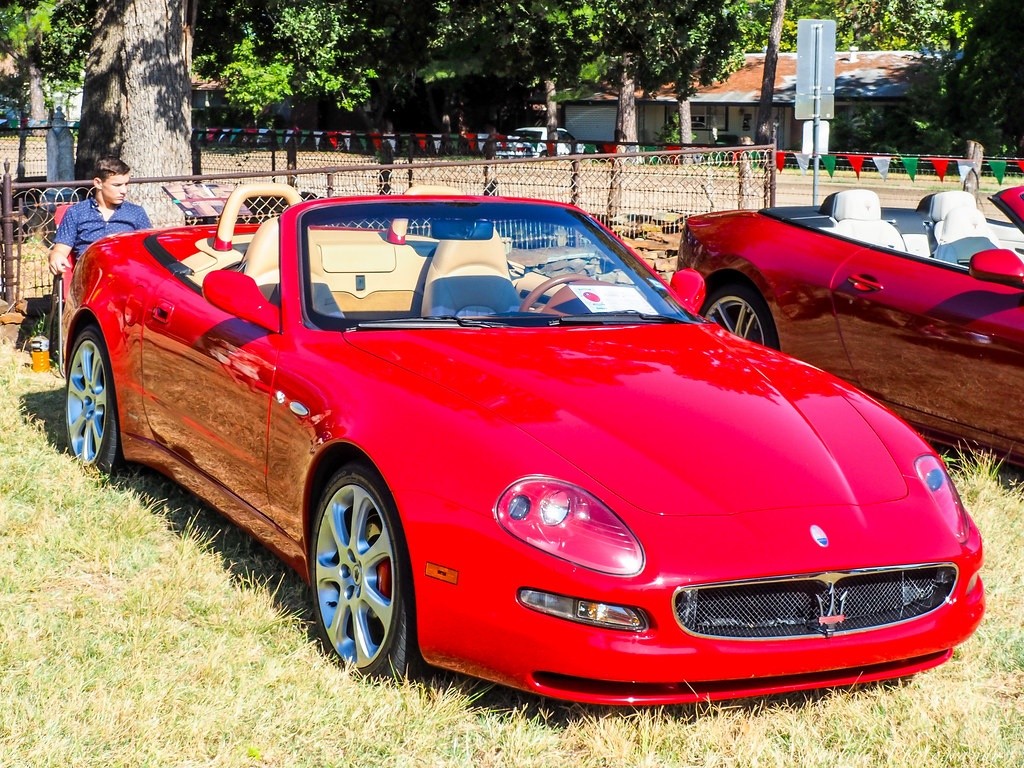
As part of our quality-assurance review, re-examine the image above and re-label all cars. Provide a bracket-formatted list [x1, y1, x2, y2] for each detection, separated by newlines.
[495, 126, 587, 160]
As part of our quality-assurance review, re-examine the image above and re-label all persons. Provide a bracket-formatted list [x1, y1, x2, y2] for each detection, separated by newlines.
[48, 154, 154, 278]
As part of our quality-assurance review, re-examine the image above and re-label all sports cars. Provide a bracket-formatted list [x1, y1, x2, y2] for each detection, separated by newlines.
[679, 171, 1024, 474]
[54, 192, 988, 716]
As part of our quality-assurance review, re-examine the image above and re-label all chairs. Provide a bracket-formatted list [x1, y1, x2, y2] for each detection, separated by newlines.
[819, 188, 908, 256]
[931, 191, 978, 244]
[48, 202, 81, 377]
[243, 217, 536, 322]
[935, 205, 1004, 269]
